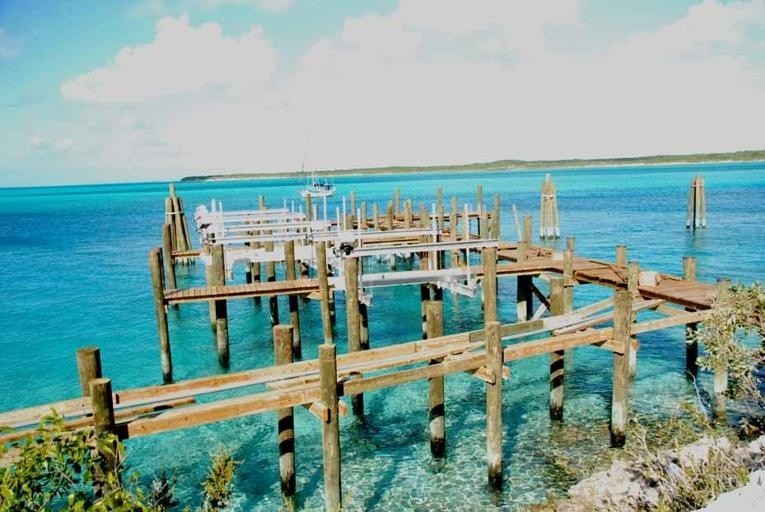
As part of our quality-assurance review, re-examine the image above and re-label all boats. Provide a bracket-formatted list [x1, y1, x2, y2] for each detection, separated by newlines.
[300, 182, 337, 198]
